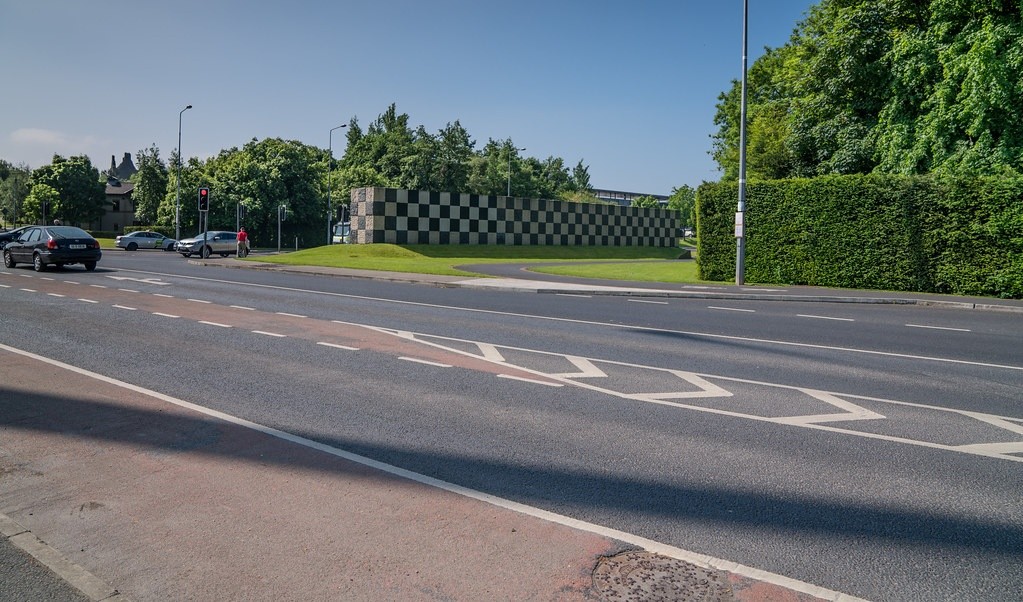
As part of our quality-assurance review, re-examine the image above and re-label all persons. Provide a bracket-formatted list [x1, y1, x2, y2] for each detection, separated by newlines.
[54, 219, 62, 226]
[237, 227, 247, 258]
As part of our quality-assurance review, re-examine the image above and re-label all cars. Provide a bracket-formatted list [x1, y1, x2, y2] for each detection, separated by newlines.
[4, 226, 103, 271]
[0, 226, 36, 247]
[115, 230, 178, 251]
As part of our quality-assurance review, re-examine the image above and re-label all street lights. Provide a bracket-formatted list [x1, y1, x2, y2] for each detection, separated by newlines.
[175, 105, 193, 239]
[327, 124, 347, 244]
[508, 148, 527, 196]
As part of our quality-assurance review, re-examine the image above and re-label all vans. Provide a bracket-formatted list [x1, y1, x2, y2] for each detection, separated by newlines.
[331, 222, 351, 244]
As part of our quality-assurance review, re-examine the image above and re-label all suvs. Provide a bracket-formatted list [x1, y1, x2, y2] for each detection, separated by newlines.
[178, 230, 249, 258]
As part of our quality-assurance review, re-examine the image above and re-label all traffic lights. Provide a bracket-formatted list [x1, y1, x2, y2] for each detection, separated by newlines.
[198, 187, 209, 211]
[282, 205, 287, 220]
